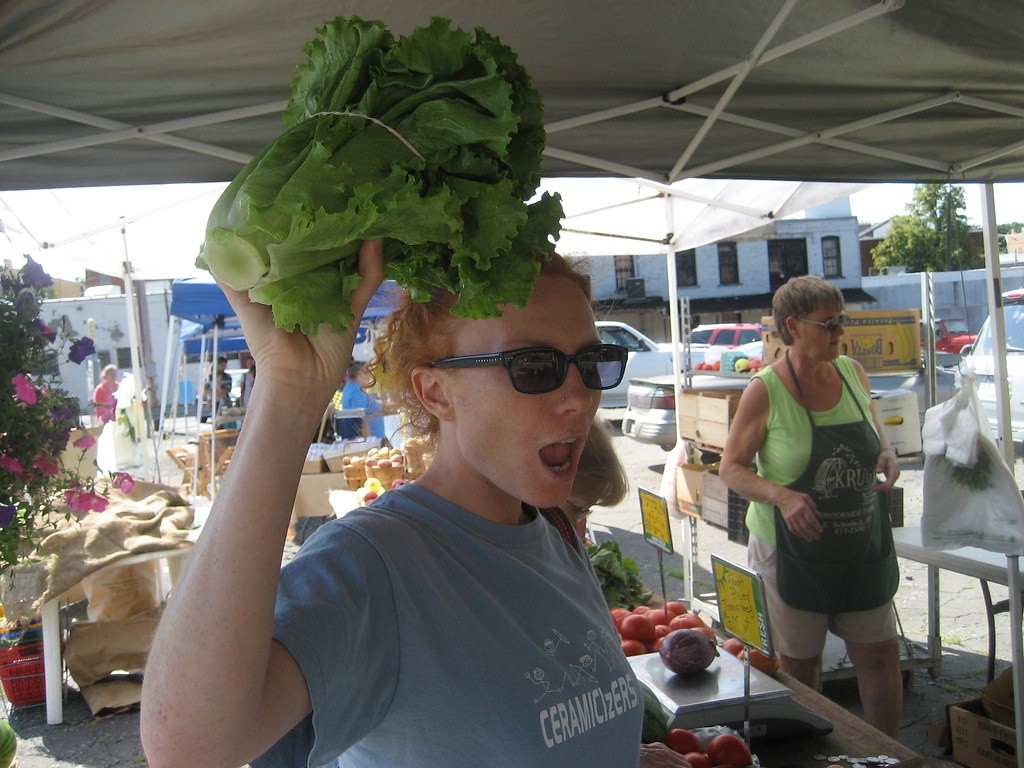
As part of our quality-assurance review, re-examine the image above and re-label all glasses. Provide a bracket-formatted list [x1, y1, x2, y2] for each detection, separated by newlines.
[797, 314, 844, 331]
[428, 341, 628, 394]
[565, 499, 594, 523]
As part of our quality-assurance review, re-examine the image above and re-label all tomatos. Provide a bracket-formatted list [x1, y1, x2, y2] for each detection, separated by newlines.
[611, 600, 778, 676]
[664, 727, 753, 768]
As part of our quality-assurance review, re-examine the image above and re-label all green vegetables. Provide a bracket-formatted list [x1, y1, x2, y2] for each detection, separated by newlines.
[584, 539, 655, 611]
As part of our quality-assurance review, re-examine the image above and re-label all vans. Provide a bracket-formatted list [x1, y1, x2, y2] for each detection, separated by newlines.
[950, 288, 1024, 448]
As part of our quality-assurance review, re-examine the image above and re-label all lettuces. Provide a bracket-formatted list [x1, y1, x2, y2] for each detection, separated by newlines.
[195, 13, 568, 339]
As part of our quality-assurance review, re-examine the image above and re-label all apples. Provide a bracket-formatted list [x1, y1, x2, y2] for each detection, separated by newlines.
[362, 477, 417, 506]
[695, 357, 762, 374]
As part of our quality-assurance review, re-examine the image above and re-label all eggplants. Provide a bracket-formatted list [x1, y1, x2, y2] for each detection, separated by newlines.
[659, 629, 720, 675]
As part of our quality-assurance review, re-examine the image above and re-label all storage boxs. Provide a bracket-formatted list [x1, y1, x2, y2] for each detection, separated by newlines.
[701, 467, 728, 530]
[760, 307, 922, 374]
[944, 699, 1018, 768]
[870, 388, 922, 457]
[980, 664, 1015, 729]
[676, 463, 720, 519]
[677, 388, 744, 449]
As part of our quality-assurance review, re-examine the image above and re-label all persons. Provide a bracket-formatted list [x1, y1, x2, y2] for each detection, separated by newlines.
[558, 419, 630, 538]
[93, 364, 121, 424]
[718, 275, 903, 742]
[239, 356, 255, 406]
[342, 362, 385, 438]
[205, 356, 232, 411]
[140, 238, 645, 768]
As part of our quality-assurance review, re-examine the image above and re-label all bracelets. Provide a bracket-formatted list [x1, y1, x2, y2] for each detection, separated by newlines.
[880, 448, 895, 453]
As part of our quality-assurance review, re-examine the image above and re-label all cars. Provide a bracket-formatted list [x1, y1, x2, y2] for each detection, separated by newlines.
[593, 319, 663, 406]
[921, 316, 979, 358]
[684, 320, 762, 369]
[616, 362, 750, 453]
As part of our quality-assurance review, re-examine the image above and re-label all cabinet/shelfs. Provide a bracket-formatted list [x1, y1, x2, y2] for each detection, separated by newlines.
[680, 272, 944, 690]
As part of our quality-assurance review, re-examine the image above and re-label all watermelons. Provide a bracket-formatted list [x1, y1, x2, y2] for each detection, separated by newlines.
[636, 678, 668, 744]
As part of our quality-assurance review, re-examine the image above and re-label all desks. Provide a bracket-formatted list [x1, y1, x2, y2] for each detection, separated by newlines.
[891, 526, 1024, 684]
[285, 472, 346, 546]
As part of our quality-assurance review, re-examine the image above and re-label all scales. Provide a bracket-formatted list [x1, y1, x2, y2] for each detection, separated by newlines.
[627, 642, 834, 742]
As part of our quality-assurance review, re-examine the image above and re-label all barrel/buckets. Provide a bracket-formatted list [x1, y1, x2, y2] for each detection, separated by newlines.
[343, 466, 366, 491]
[366, 466, 404, 491]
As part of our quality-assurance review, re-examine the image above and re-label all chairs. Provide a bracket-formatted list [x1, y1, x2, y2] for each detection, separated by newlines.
[165, 427, 239, 503]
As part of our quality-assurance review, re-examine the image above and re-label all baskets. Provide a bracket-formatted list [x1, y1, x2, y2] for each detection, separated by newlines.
[0, 600, 64, 707]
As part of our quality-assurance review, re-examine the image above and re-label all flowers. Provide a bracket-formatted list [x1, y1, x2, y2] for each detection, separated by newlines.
[0, 252, 136, 595]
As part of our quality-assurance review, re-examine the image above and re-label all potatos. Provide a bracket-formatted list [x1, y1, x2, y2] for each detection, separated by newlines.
[342, 445, 403, 469]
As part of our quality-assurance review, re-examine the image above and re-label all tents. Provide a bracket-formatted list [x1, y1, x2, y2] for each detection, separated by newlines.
[159, 273, 399, 500]
